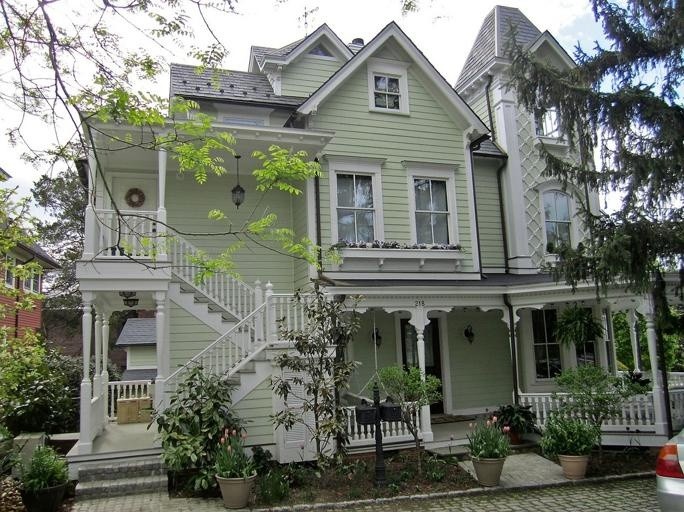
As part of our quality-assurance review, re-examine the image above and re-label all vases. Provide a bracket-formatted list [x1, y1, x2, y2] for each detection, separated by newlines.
[214, 473, 257, 509]
[473, 458, 505, 486]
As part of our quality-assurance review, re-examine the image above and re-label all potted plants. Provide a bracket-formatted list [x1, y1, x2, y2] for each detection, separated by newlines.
[542, 243, 559, 265]
[553, 302, 606, 347]
[488, 403, 536, 443]
[539, 409, 602, 480]
[16, 445, 68, 512]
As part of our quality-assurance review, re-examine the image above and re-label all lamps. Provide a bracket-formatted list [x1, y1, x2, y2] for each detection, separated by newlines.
[231, 155, 245, 211]
[465, 325, 475, 344]
[123, 297, 140, 307]
[355, 382, 403, 489]
[372, 327, 381, 346]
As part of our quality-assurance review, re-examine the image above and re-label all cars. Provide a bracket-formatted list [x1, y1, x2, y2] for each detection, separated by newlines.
[655, 427, 684, 512]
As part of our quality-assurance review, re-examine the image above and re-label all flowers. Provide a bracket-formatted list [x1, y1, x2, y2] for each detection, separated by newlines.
[460, 413, 510, 461]
[209, 427, 263, 479]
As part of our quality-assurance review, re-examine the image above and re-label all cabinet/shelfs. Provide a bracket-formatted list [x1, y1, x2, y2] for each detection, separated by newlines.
[115, 398, 150, 424]
